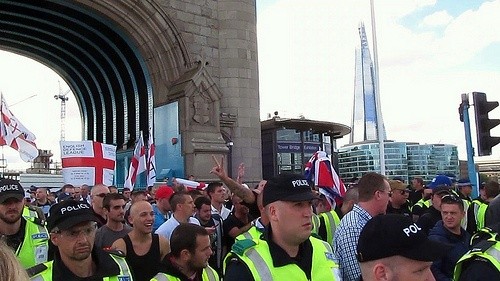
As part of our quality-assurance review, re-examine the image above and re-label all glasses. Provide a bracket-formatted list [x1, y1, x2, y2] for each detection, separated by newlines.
[442, 195, 460, 202]
[393, 190, 405, 195]
[93, 193, 110, 197]
[374, 190, 393, 197]
[53, 223, 99, 237]
[236, 200, 245, 205]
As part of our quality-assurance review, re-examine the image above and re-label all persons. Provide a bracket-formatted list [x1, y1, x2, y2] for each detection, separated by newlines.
[0, 155, 270, 281]
[386, 175, 500, 281]
[333, 172, 390, 281]
[223, 174, 343, 281]
[357, 215, 456, 281]
[312, 185, 358, 245]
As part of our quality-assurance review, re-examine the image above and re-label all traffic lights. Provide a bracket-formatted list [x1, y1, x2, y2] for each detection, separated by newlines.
[470, 91, 500, 157]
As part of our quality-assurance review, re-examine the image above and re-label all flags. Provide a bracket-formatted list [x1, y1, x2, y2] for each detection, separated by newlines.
[147, 142, 156, 186]
[0, 95, 38, 163]
[124, 138, 147, 191]
[324, 160, 347, 198]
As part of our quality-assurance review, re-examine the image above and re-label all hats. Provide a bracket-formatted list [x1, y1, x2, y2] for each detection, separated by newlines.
[432, 185, 452, 196]
[388, 180, 410, 191]
[354, 213, 454, 262]
[479, 181, 486, 188]
[48, 200, 103, 229]
[429, 175, 452, 189]
[156, 186, 175, 199]
[0, 179, 25, 203]
[456, 178, 475, 185]
[262, 172, 322, 206]
